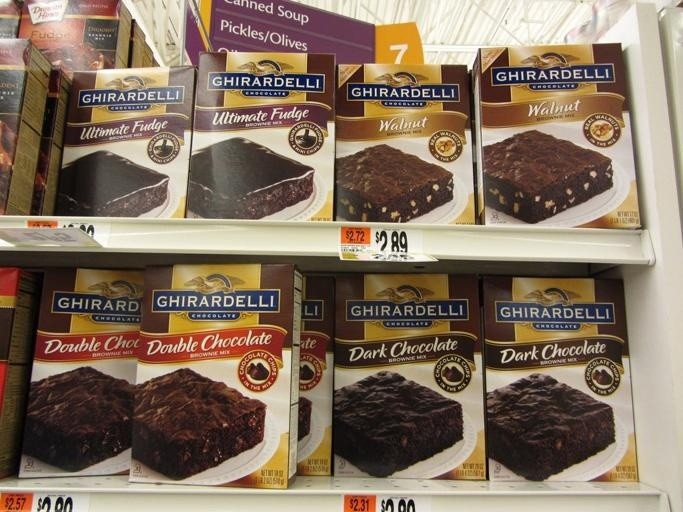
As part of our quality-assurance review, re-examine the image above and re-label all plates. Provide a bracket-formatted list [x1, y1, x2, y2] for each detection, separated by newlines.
[485, 158, 632, 227]
[300, 401, 327, 461]
[341, 167, 468, 221]
[344, 416, 479, 477]
[211, 175, 327, 222]
[61, 173, 178, 217]
[501, 403, 631, 482]
[136, 408, 280, 483]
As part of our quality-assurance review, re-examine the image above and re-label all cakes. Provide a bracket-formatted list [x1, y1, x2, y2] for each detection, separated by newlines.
[186, 137, 315, 219]
[56, 151, 170, 217]
[298, 397, 313, 440]
[333, 371, 464, 477]
[483, 130, 614, 223]
[132, 369, 267, 481]
[25, 367, 135, 472]
[336, 144, 454, 221]
[486, 372, 616, 481]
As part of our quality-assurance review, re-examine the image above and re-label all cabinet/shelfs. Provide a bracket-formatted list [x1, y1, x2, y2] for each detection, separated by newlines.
[0, 3, 682, 512]
